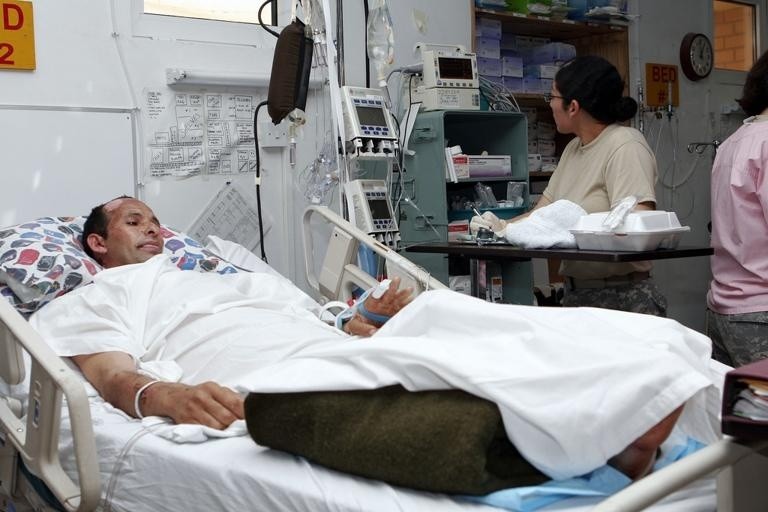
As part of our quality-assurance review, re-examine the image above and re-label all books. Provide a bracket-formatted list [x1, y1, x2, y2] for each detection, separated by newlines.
[732, 378, 768, 421]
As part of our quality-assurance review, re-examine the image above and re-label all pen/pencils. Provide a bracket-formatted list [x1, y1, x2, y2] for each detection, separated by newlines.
[470, 205, 493, 231]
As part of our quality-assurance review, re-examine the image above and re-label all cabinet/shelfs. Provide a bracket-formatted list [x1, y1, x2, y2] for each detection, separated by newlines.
[470, 2, 630, 202]
[403, 107, 534, 310]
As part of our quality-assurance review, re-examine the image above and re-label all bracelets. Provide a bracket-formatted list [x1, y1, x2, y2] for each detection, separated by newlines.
[135, 380, 160, 419]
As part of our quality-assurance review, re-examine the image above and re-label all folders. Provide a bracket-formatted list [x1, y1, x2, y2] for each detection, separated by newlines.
[722, 358, 768, 425]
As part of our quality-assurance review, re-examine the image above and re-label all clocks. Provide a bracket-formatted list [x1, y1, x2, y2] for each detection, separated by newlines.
[680, 31, 713, 82]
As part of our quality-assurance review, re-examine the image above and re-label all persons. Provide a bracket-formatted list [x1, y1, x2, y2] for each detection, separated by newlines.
[469, 55, 668, 318]
[704, 49, 768, 368]
[28, 195, 684, 482]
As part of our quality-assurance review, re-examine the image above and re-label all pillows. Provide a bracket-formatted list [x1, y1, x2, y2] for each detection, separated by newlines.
[0, 217, 249, 316]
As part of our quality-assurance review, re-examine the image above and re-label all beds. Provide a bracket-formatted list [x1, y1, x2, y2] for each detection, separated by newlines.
[1, 204, 768, 510]
